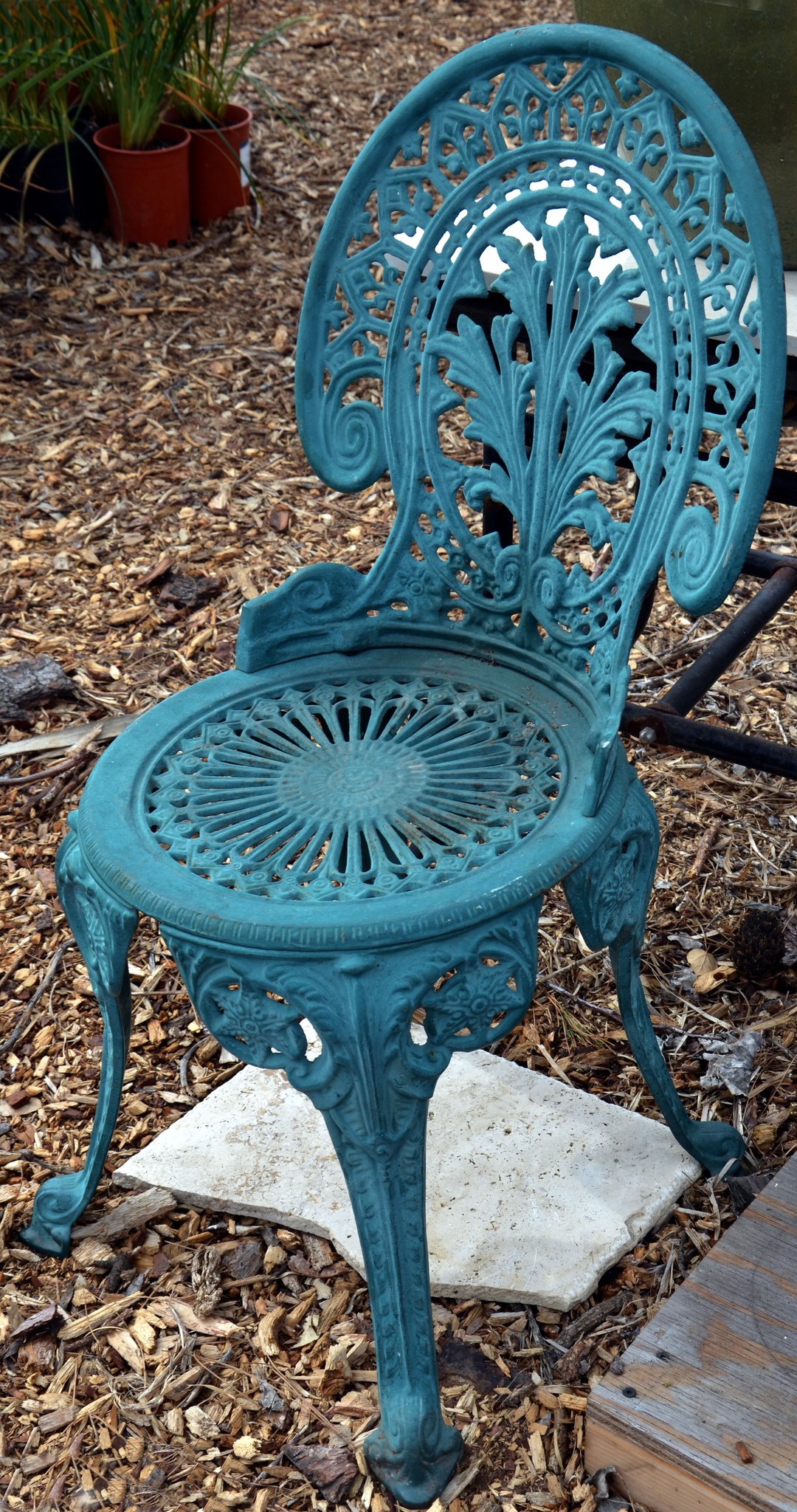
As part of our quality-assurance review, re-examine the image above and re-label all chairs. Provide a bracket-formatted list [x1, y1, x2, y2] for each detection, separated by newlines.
[16, 21, 787, 1506]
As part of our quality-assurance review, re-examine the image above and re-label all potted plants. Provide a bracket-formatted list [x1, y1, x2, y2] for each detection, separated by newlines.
[0, 0, 307, 247]
